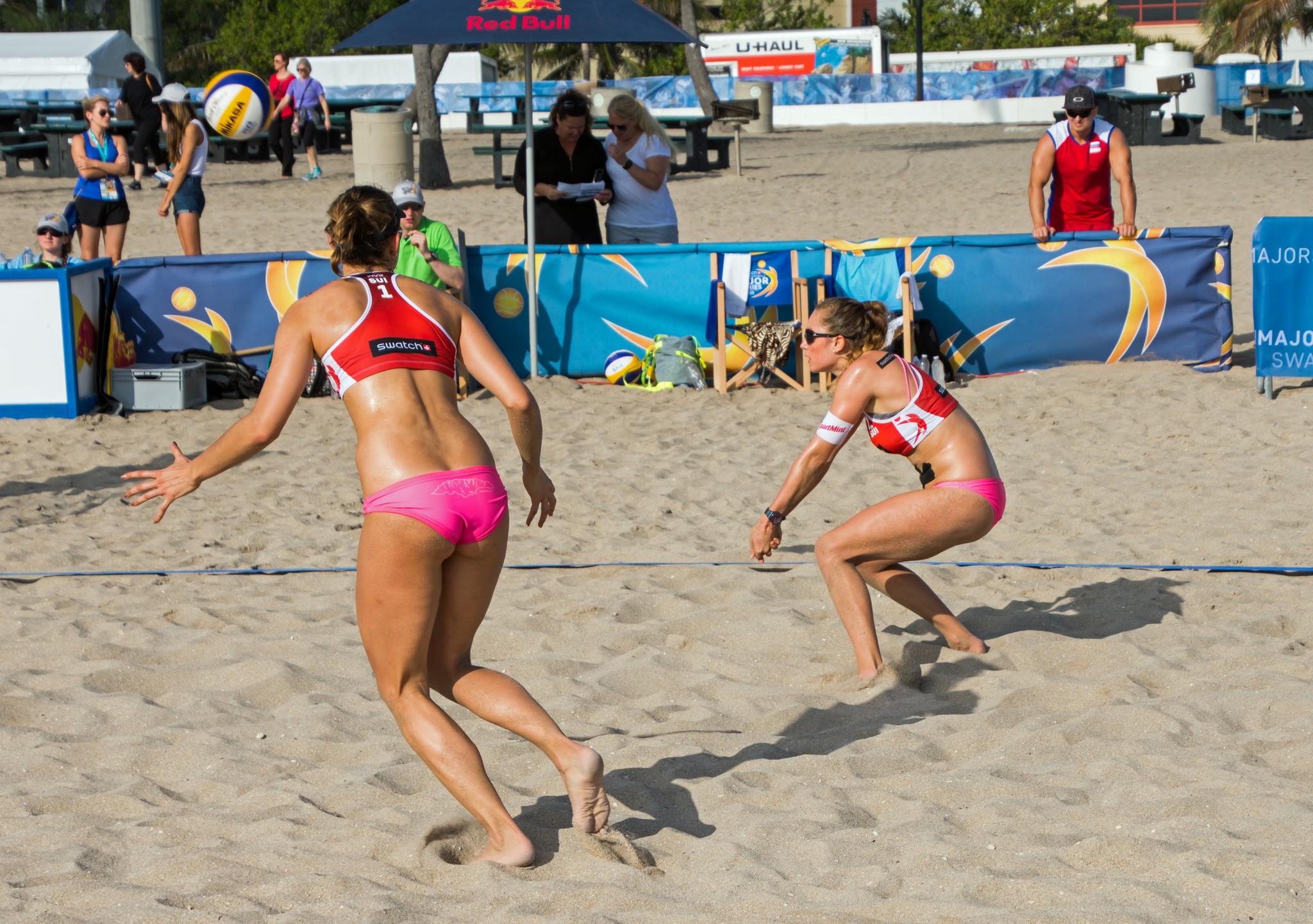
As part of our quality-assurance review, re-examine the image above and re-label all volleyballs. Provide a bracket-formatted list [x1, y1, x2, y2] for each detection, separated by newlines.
[642, 348, 657, 384]
[202, 70, 274, 141]
[604, 349, 642, 386]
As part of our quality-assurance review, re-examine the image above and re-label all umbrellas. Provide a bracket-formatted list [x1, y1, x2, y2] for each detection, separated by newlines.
[331, 0, 708, 378]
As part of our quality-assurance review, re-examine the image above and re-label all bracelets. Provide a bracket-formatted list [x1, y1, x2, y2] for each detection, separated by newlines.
[623, 159, 633, 170]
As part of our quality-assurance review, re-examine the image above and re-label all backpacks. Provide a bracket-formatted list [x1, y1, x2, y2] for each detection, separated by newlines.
[894, 319, 954, 383]
[301, 358, 331, 398]
[623, 334, 706, 392]
[171, 348, 265, 400]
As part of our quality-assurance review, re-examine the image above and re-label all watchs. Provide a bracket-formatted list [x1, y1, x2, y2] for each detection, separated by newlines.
[764, 507, 785, 525]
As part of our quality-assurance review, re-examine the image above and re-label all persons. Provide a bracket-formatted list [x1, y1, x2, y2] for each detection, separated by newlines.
[151, 82, 208, 256]
[748, 297, 1008, 684]
[70, 95, 130, 265]
[513, 89, 615, 246]
[271, 58, 331, 182]
[115, 53, 169, 191]
[391, 179, 464, 292]
[601, 94, 679, 244]
[1027, 85, 1137, 241]
[268, 53, 296, 179]
[119, 184, 612, 866]
[0, 214, 85, 270]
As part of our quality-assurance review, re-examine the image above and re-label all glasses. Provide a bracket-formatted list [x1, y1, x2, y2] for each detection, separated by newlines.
[399, 202, 422, 211]
[1066, 108, 1093, 118]
[804, 329, 857, 345]
[393, 226, 403, 240]
[37, 227, 63, 237]
[98, 108, 115, 118]
[606, 122, 628, 131]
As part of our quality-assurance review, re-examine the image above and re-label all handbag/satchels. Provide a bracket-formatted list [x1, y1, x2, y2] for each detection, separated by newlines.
[291, 109, 301, 136]
[63, 201, 80, 239]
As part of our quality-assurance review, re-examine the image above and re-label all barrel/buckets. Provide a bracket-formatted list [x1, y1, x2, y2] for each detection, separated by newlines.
[349, 105, 415, 197]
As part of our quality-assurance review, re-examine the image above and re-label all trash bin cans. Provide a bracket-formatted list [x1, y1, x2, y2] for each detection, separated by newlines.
[351, 104, 416, 193]
[590, 86, 638, 117]
[734, 79, 774, 134]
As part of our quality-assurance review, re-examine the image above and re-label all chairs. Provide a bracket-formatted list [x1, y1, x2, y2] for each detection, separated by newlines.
[817, 247, 916, 393]
[705, 250, 810, 394]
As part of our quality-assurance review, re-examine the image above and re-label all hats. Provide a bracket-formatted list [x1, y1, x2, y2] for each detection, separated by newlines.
[152, 83, 191, 103]
[392, 179, 423, 205]
[394, 207, 406, 218]
[37, 212, 68, 233]
[1062, 84, 1098, 109]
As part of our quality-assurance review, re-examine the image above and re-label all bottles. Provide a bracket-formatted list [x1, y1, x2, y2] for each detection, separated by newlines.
[931, 356, 946, 389]
[685, 360, 708, 392]
[919, 354, 930, 375]
[912, 355, 921, 368]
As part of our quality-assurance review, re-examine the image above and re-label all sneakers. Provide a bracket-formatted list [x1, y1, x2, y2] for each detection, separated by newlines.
[149, 181, 167, 189]
[125, 179, 142, 191]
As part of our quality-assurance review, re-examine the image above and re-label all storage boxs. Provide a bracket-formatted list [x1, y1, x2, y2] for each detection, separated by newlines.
[110, 362, 207, 411]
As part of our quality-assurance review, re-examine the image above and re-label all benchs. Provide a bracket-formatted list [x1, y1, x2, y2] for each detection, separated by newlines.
[1260, 108, 1293, 140]
[0, 117, 351, 177]
[1053, 111, 1104, 124]
[454, 111, 551, 114]
[596, 136, 734, 170]
[472, 146, 521, 189]
[1172, 113, 1205, 144]
[1220, 104, 1247, 136]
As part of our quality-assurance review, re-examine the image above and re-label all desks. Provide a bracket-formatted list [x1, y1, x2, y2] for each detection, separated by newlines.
[538, 116, 713, 175]
[1093, 90, 1173, 146]
[471, 122, 606, 189]
[0, 99, 406, 144]
[463, 95, 559, 134]
[1240, 84, 1313, 139]
[30, 120, 134, 179]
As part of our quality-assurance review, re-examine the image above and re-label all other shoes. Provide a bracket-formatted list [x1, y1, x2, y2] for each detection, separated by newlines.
[302, 171, 318, 181]
[313, 165, 323, 178]
